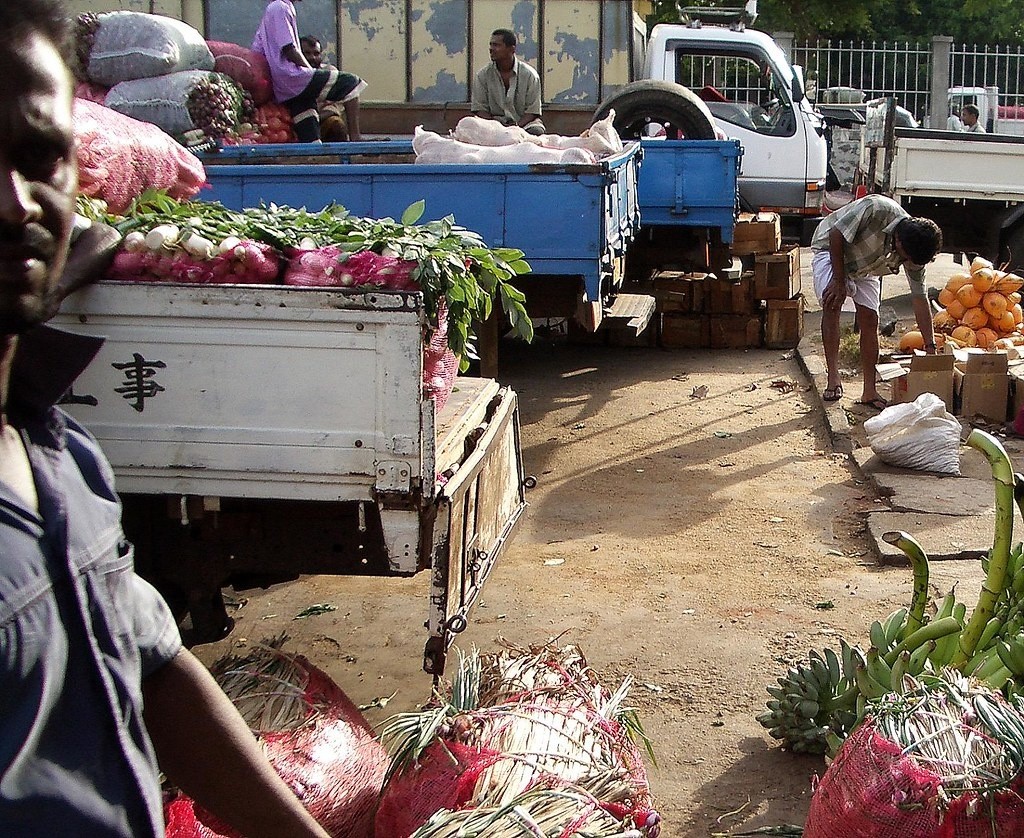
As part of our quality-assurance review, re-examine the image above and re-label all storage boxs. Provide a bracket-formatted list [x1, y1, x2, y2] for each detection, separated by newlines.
[587, 211, 804, 349]
[873, 350, 1024, 425]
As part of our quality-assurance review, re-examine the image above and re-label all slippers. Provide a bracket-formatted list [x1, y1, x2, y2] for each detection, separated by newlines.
[822, 386, 843, 401]
[855, 395, 889, 412]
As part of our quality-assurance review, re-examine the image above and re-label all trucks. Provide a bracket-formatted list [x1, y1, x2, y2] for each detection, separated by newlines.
[609, 133, 747, 276]
[348, 5, 837, 251]
[160, 139, 645, 332]
[855, 94, 1024, 292]
[24, 281, 538, 710]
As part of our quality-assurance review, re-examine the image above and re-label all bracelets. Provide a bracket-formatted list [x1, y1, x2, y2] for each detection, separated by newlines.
[925, 342, 936, 348]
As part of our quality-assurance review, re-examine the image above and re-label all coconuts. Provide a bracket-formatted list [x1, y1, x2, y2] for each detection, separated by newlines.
[899, 257, 1024, 351]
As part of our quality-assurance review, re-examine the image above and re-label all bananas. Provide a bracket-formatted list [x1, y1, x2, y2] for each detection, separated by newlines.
[754, 541, 1024, 763]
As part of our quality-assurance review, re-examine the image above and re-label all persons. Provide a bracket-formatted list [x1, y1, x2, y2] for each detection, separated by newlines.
[812, 195, 943, 408]
[947, 114, 961, 131]
[0, 1, 331, 838]
[251, 0, 368, 143]
[300, 35, 349, 141]
[962, 104, 986, 133]
[472, 28, 545, 136]
